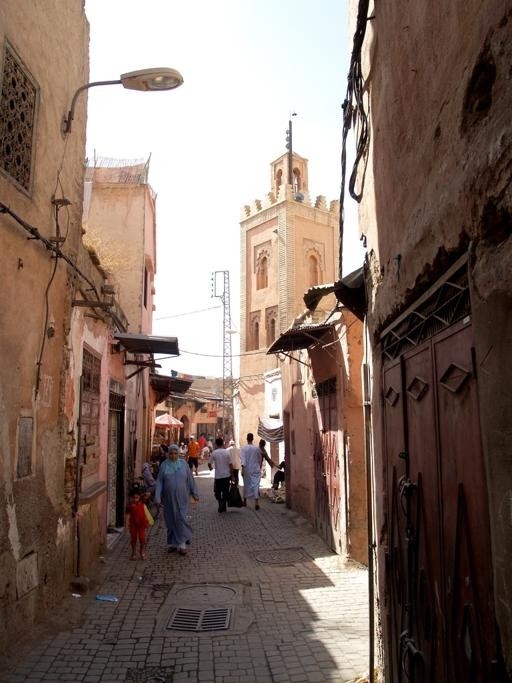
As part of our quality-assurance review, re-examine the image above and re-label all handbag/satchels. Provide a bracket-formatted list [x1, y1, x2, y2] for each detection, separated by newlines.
[227, 484, 243, 508]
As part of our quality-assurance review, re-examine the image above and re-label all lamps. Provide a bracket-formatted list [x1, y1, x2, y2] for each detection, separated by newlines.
[61, 63, 184, 139]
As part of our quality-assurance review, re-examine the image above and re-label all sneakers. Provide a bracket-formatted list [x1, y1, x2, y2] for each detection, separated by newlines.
[255, 504, 260, 511]
[130, 552, 147, 561]
[167, 542, 187, 553]
[218, 502, 226, 513]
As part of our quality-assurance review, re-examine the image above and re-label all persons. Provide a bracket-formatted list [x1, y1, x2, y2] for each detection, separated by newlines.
[184, 435, 200, 476]
[197, 426, 234, 465]
[271, 457, 286, 490]
[157, 439, 168, 467]
[123, 488, 155, 560]
[240, 432, 263, 510]
[257, 439, 275, 496]
[179, 441, 188, 462]
[208, 437, 233, 513]
[154, 444, 199, 556]
[227, 441, 242, 486]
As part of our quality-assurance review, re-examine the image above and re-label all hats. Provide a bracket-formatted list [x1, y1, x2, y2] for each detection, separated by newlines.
[190, 436, 194, 439]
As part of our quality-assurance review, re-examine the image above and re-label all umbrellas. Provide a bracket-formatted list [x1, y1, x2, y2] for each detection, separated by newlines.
[155, 412, 184, 443]
